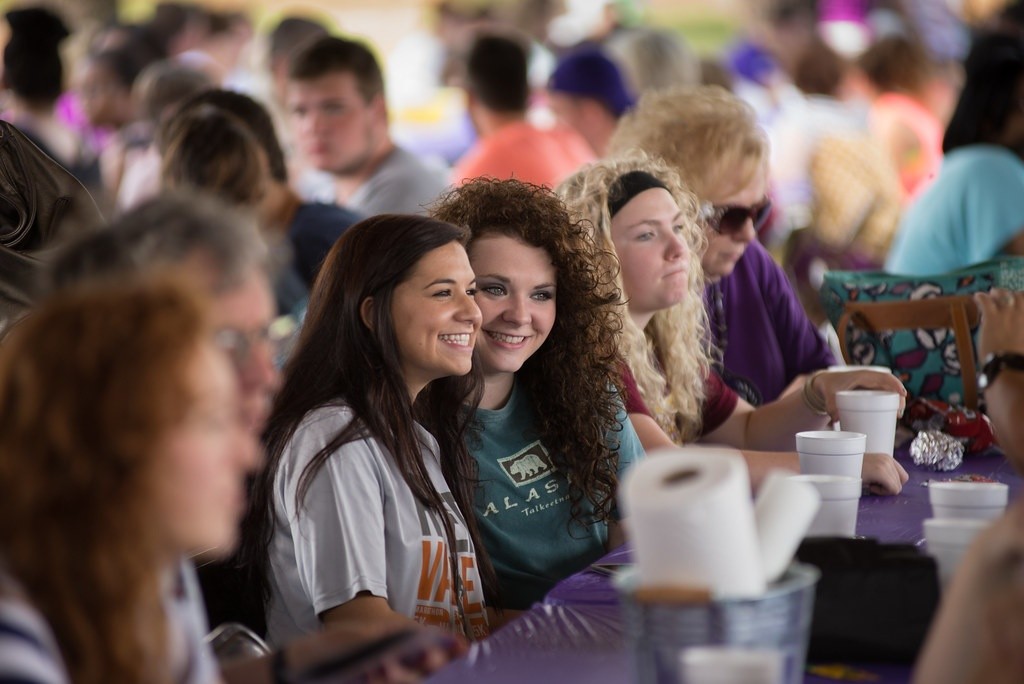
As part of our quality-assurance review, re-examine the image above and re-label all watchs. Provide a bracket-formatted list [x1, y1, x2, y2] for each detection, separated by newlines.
[976, 350, 1024, 390]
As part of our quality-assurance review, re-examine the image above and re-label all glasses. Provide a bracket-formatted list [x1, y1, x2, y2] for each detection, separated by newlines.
[706, 194, 773, 236]
[217, 314, 300, 355]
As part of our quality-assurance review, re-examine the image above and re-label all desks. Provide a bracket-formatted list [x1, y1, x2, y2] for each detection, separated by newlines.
[416, 452, 1024, 684]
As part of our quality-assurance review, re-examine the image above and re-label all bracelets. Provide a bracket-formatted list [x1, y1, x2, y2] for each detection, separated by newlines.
[801, 370, 828, 415]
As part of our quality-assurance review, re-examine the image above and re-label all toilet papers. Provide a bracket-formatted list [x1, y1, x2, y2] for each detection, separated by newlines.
[752, 467, 824, 584]
[621, 446, 766, 599]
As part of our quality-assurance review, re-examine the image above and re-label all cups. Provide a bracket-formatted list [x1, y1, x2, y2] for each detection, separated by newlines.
[795, 431, 866, 475]
[836, 390, 899, 458]
[610, 561, 822, 684]
[800, 474, 862, 538]
[929, 481, 1009, 519]
[923, 519, 990, 585]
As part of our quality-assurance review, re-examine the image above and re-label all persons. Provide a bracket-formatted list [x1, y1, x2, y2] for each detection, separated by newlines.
[973, 286, 1024, 481]
[911, 487, 1024, 684]
[551, 152, 908, 497]
[444, 34, 599, 196]
[438, 0, 1024, 195]
[547, 50, 636, 158]
[46, 193, 285, 473]
[0, 273, 243, 684]
[243, 215, 491, 684]
[0, 0, 364, 369]
[285, 34, 454, 217]
[885, 32, 1024, 276]
[428, 178, 647, 635]
[602, 85, 837, 408]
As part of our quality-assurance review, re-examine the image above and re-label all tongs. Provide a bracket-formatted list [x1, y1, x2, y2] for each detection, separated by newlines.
[590, 564, 626, 576]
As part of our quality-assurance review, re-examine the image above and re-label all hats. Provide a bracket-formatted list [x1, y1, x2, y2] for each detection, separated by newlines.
[547, 51, 627, 116]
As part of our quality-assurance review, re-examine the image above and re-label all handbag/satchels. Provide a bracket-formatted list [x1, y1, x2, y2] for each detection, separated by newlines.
[796, 536, 941, 665]
[820, 255, 1024, 412]
